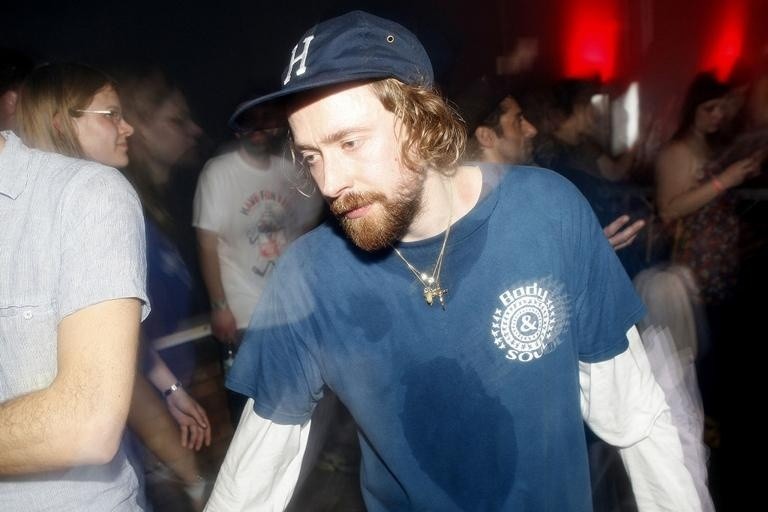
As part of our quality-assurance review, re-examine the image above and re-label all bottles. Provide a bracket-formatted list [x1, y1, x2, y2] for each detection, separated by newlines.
[222, 339, 235, 379]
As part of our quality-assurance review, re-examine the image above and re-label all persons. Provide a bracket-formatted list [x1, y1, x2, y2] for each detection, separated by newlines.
[466, 69, 766, 512]
[0, 62, 361, 512]
[202, 12, 705, 511]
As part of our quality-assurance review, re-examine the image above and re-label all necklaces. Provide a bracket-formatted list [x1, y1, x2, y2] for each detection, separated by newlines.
[392, 170, 454, 309]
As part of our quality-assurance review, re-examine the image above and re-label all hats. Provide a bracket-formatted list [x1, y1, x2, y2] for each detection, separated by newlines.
[229, 11, 435, 132]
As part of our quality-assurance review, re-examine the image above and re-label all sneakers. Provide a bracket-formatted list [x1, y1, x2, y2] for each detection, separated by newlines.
[313, 449, 362, 477]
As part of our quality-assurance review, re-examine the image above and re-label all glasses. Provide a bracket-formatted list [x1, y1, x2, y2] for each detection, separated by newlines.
[77, 107, 119, 120]
